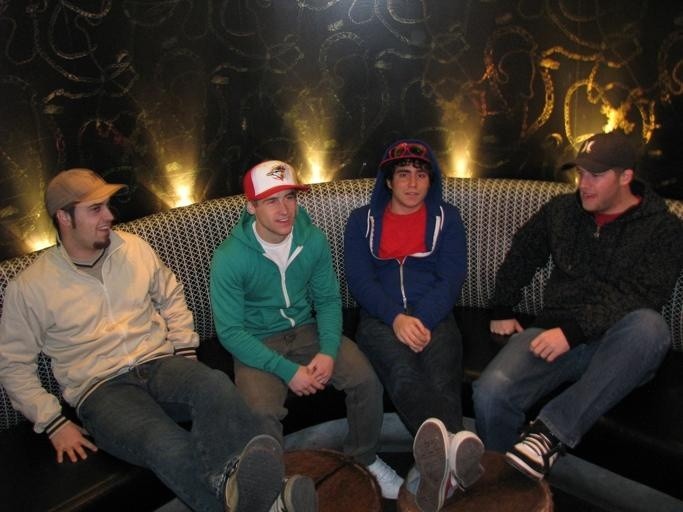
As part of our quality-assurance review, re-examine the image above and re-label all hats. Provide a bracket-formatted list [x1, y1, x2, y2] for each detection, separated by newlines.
[559, 133, 637, 173]
[44, 167, 130, 216]
[243, 159, 308, 202]
[380, 143, 432, 167]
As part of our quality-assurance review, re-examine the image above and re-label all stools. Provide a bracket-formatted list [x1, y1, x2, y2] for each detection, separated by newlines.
[399, 450, 552, 512]
[281, 448, 380, 512]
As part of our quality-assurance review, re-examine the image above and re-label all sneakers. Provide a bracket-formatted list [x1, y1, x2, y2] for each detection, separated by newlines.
[268, 474, 319, 512]
[502, 415, 565, 481]
[366, 453, 405, 500]
[222, 434, 286, 512]
[413, 416, 456, 512]
[446, 430, 486, 494]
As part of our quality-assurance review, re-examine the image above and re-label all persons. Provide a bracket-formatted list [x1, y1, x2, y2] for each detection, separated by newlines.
[469, 130, 683, 482]
[209, 156, 409, 505]
[0, 166, 321, 512]
[340, 142, 486, 511]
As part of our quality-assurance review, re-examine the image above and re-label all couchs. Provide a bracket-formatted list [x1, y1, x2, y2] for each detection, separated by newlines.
[0, 176, 681, 512]
